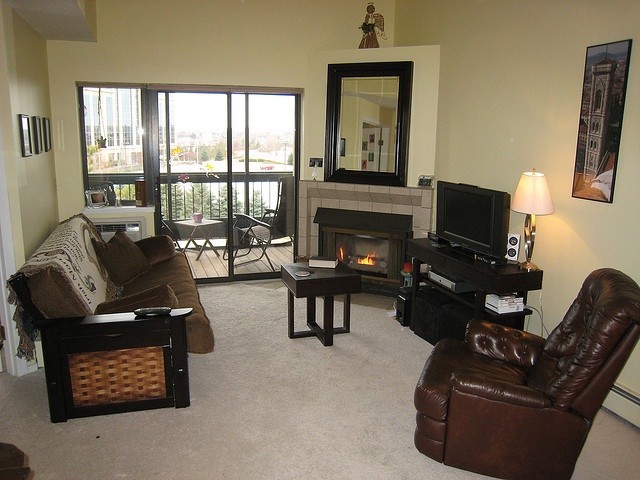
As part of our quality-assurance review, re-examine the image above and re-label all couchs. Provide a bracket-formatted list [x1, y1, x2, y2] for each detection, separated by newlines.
[414, 268, 640, 480]
[5, 212, 215, 423]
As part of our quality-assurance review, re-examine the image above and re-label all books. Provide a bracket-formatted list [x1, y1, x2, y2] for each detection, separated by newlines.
[307, 255, 339, 269]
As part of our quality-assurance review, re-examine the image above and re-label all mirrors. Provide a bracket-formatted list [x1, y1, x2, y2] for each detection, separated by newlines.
[324, 61, 414, 188]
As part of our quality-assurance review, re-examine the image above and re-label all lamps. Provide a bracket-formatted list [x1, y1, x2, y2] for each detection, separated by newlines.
[510, 168, 555, 272]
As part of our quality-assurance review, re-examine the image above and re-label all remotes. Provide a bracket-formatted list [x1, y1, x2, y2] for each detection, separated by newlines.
[135, 307, 171, 316]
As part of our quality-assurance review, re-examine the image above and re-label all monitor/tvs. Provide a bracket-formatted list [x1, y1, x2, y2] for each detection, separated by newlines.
[436, 181, 510, 258]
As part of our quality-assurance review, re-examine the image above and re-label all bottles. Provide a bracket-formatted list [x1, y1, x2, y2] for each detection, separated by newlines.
[135, 177, 147, 207]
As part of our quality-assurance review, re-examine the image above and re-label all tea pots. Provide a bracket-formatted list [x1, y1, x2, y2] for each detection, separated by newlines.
[102, 181, 116, 206]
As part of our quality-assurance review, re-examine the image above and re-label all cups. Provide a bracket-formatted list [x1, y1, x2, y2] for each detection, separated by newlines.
[192, 213, 203, 223]
[114, 198, 123, 208]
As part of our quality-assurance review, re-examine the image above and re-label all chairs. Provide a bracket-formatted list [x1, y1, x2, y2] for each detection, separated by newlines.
[223, 176, 295, 273]
[91, 181, 182, 252]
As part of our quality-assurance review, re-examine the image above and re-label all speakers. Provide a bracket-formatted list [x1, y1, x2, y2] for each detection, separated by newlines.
[507, 233, 521, 264]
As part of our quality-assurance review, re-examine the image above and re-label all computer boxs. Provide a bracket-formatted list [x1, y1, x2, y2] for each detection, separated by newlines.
[396, 286, 430, 326]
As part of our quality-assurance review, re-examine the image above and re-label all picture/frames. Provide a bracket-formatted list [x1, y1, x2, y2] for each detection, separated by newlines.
[340, 137, 347, 157]
[572, 38, 633, 203]
[19, 114, 32, 157]
[33, 116, 43, 154]
[43, 117, 51, 152]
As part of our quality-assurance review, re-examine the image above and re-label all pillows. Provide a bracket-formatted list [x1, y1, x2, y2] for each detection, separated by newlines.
[91, 230, 152, 287]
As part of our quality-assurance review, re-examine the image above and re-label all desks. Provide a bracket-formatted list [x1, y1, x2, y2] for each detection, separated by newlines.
[405, 238, 544, 347]
[173, 220, 223, 261]
